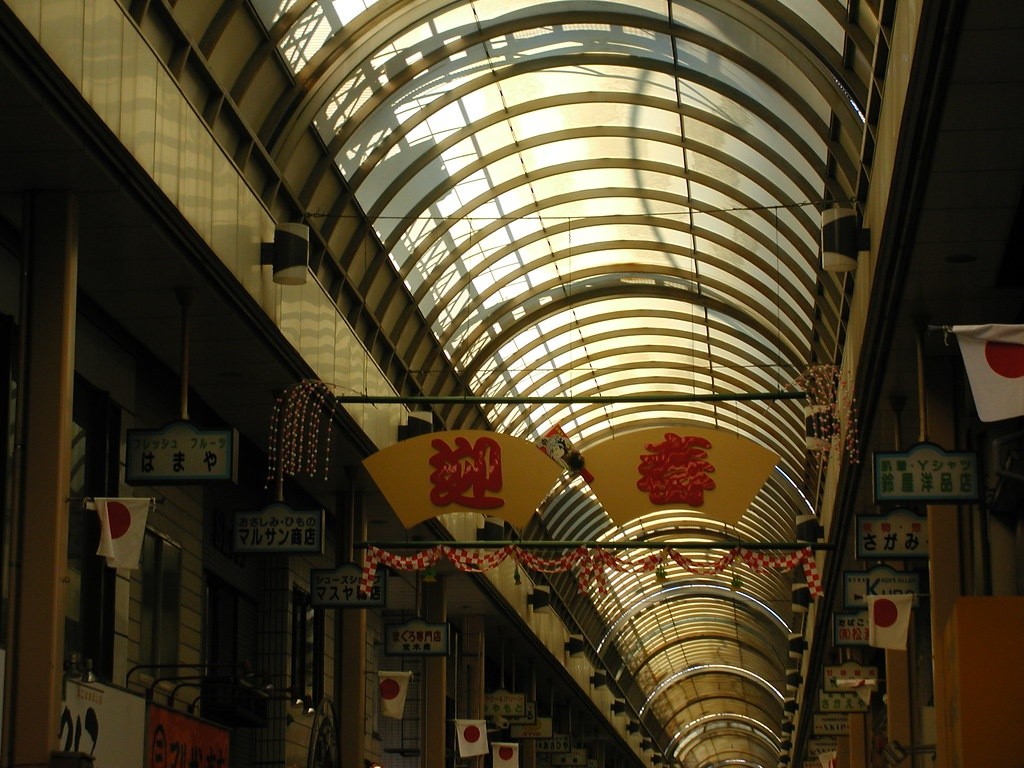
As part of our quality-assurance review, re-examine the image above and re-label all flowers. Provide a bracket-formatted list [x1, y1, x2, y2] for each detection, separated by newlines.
[265, 380, 377, 491]
[764, 363, 860, 470]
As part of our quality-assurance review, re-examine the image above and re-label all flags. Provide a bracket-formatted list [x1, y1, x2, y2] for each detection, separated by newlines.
[378, 670, 411, 721]
[454, 719, 490, 758]
[835, 677, 877, 706]
[818, 751, 837, 768]
[93, 497, 150, 570]
[862, 594, 913, 651]
[491, 741, 520, 768]
[951, 323, 1024, 422]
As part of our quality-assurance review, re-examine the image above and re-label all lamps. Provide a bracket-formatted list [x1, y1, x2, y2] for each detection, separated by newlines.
[82, 659, 97, 683]
[795, 514, 824, 545]
[792, 583, 815, 613]
[151, 672, 274, 700]
[192, 695, 315, 715]
[261, 224, 309, 286]
[780, 738, 792, 753]
[611, 698, 625, 717]
[383, 748, 421, 757]
[782, 720, 795, 737]
[784, 697, 799, 716]
[565, 634, 584, 658]
[640, 737, 652, 752]
[126, 661, 255, 689]
[590, 670, 606, 690]
[789, 633, 808, 659]
[882, 739, 936, 768]
[822, 208, 870, 273]
[476, 517, 504, 550]
[626, 719, 640, 736]
[785, 669, 803, 691]
[170, 684, 304, 706]
[63, 654, 83, 678]
[805, 406, 841, 451]
[652, 753, 670, 768]
[398, 411, 432, 440]
[777, 754, 791, 768]
[527, 585, 550, 613]
